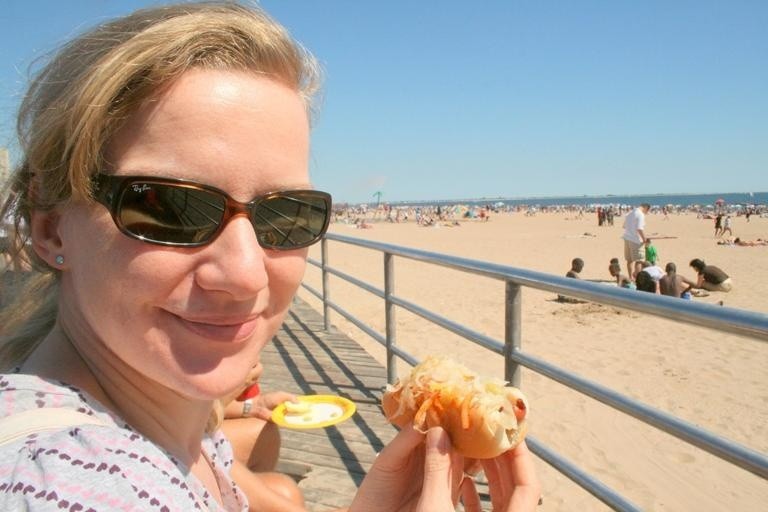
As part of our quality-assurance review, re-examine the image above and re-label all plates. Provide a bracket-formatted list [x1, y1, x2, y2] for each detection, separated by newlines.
[269, 394, 358, 431]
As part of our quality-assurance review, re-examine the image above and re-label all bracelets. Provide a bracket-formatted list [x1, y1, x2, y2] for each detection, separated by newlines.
[241, 399, 254, 416]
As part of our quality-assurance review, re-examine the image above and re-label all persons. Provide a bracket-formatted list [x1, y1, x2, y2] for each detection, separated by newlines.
[606, 255, 734, 303]
[334, 203, 477, 229]
[618, 201, 650, 277]
[557, 256, 589, 304]
[652, 204, 714, 220]
[214, 353, 307, 511]
[614, 204, 636, 216]
[479, 201, 596, 222]
[0, 0, 542, 512]
[598, 204, 615, 226]
[713, 205, 766, 246]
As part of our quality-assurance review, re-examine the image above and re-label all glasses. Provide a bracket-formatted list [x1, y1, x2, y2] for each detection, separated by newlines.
[89, 173, 331, 251]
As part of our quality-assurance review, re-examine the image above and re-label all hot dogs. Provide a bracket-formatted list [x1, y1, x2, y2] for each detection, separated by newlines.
[381, 354, 530, 460]
